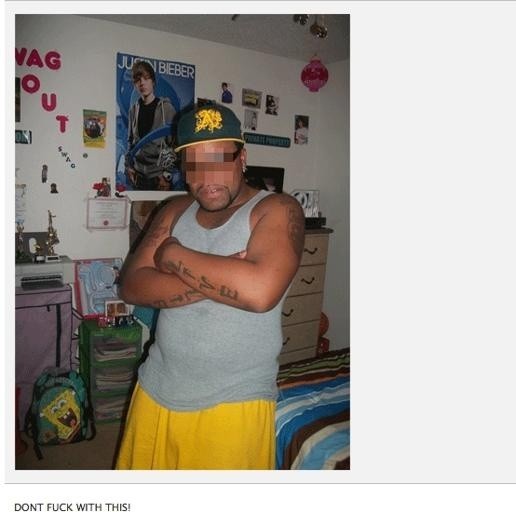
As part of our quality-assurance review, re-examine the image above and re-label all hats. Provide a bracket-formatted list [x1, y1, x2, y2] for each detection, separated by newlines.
[170, 103, 246, 154]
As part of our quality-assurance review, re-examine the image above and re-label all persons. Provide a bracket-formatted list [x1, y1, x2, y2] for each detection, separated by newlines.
[97, 177, 110, 198]
[89, 122, 98, 138]
[266, 99, 278, 115]
[221, 83, 233, 103]
[97, 118, 107, 138]
[111, 96, 309, 469]
[296, 118, 309, 145]
[124, 60, 179, 190]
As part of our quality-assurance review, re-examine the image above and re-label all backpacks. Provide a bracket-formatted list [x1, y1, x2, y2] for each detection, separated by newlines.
[31, 368, 90, 445]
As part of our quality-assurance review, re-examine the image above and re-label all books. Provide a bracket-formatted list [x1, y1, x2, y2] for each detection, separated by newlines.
[92, 344, 138, 421]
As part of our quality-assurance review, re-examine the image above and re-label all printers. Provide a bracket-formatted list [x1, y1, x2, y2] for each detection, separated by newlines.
[15, 254, 75, 291]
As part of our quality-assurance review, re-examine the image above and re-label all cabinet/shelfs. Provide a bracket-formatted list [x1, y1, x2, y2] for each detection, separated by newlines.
[277, 227, 334, 367]
[78, 317, 143, 424]
[16, 283, 84, 431]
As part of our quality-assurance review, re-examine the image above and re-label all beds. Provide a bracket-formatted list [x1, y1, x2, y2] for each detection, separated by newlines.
[273, 347, 350, 470]
